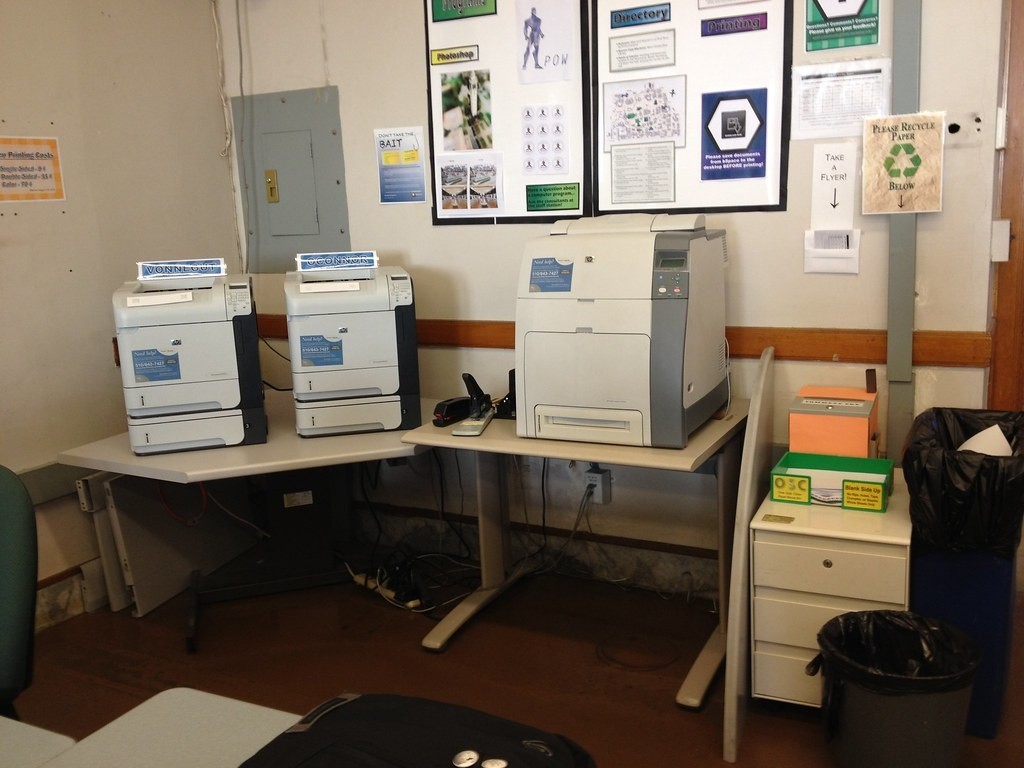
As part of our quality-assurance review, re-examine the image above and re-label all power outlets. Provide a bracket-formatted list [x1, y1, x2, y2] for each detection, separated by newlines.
[586, 468, 610, 505]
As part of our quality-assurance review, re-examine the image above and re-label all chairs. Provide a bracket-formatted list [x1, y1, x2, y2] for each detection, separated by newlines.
[0, 465, 38, 724]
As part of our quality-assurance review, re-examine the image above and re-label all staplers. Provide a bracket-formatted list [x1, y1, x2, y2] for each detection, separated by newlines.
[432, 397, 475, 428]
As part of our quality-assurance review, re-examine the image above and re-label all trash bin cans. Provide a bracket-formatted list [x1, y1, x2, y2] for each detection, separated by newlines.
[906, 405, 1024, 743]
[816, 609, 983, 768]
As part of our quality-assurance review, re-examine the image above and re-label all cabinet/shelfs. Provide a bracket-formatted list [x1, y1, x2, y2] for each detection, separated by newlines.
[750, 467, 915, 727]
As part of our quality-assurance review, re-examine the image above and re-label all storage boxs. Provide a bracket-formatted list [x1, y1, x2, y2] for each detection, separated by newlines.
[765, 384, 894, 514]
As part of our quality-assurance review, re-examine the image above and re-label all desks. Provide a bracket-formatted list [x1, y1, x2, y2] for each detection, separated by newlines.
[59, 382, 453, 606]
[38, 686, 306, 767]
[399, 394, 750, 714]
